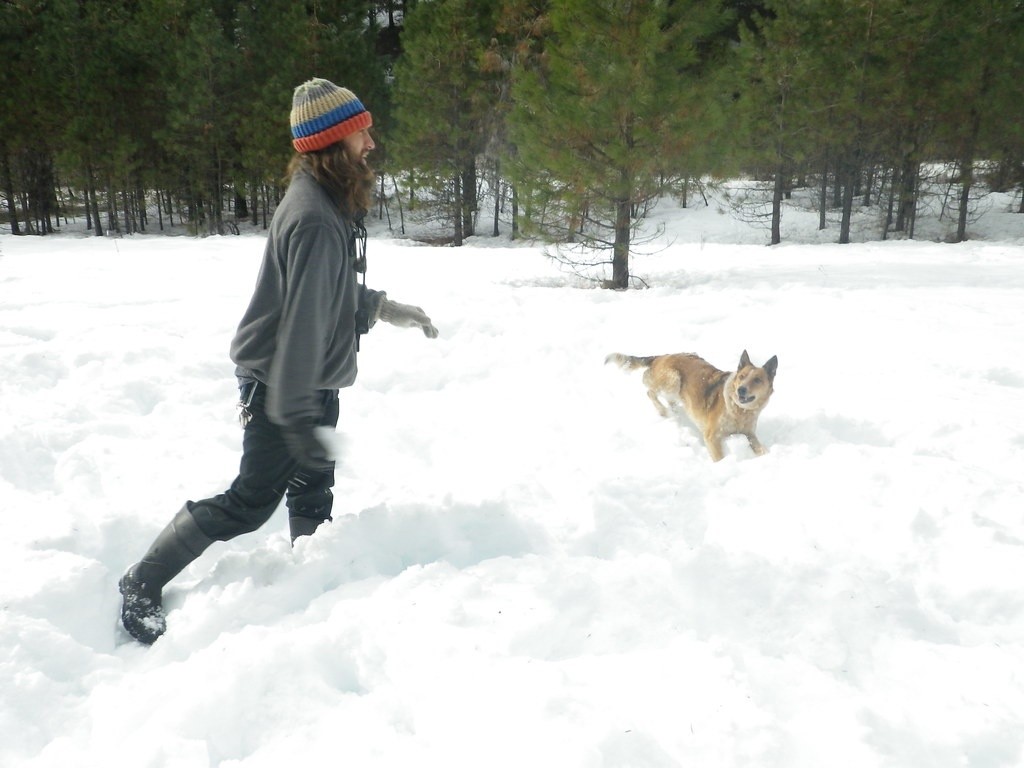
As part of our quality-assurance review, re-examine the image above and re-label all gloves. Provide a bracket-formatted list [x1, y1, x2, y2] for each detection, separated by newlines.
[281, 419, 335, 474]
[373, 294, 439, 339]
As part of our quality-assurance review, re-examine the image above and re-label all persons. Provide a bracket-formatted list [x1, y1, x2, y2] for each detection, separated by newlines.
[117, 78, 437, 644]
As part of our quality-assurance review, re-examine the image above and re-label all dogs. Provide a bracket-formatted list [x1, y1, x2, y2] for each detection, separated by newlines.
[598, 345, 778, 461]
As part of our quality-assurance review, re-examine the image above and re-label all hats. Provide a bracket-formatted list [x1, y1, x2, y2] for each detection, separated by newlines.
[290, 77, 372, 154]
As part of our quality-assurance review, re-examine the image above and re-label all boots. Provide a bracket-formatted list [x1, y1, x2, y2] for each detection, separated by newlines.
[290, 516, 332, 548]
[119, 499, 214, 645]
